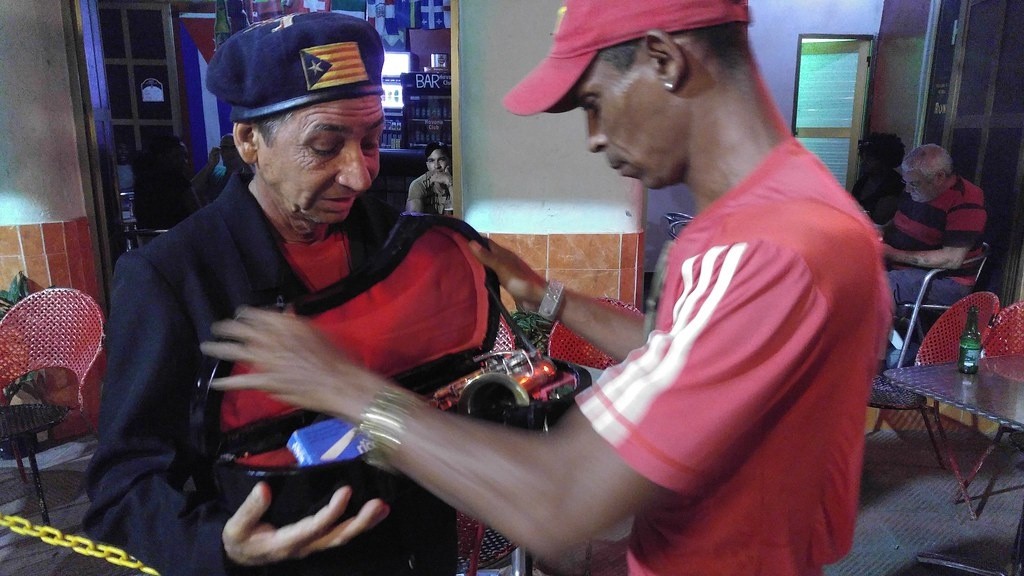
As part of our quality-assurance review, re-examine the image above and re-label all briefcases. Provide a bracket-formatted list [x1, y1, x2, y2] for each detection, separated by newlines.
[189, 211, 593, 533]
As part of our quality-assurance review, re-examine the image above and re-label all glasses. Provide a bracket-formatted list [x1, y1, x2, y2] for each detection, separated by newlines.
[900, 175, 937, 191]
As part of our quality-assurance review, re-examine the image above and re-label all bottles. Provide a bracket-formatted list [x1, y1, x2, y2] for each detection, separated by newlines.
[957, 306, 981, 373]
[214, 0, 230, 53]
[414, 99, 452, 145]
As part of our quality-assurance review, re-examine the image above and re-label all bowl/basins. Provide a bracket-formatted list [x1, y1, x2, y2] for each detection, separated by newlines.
[423, 66, 449, 74]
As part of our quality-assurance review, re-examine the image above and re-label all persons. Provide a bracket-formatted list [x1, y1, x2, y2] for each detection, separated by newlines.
[116, 140, 135, 190]
[80, 11, 481, 576]
[190, 133, 243, 203]
[134, 134, 200, 229]
[851, 133, 906, 223]
[200, 0, 893, 576]
[872, 143, 987, 306]
[407, 141, 453, 214]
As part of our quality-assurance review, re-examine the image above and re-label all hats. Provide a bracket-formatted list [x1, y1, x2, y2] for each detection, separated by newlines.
[206, 12, 386, 120]
[501, 0, 748, 116]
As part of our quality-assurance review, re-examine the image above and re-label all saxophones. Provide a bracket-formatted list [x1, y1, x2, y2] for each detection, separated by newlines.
[430, 343, 559, 418]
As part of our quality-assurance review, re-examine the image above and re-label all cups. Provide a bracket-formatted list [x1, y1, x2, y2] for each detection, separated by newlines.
[444, 208, 453, 216]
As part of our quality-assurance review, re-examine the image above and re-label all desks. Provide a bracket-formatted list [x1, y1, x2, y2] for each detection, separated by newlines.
[0, 404, 71, 527]
[883, 354, 1024, 522]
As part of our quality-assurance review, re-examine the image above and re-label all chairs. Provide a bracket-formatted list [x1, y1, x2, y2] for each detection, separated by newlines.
[0, 286, 108, 483]
[444, 299, 647, 576]
[884, 241, 992, 371]
[866, 291, 1024, 524]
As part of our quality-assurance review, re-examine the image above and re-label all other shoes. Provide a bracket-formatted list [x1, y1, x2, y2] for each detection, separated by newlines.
[885, 344, 920, 371]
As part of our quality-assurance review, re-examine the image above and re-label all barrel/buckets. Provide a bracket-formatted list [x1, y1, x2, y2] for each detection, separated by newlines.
[431, 53, 448, 67]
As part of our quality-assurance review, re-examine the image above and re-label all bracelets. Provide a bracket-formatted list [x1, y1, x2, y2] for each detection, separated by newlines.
[357, 383, 423, 475]
[537, 279, 566, 321]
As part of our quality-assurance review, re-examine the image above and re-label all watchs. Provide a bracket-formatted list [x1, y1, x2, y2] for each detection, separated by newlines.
[447, 176, 453, 188]
